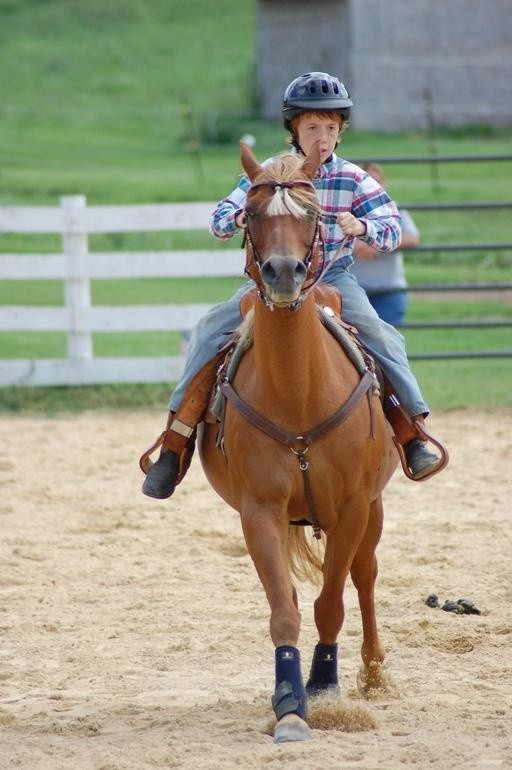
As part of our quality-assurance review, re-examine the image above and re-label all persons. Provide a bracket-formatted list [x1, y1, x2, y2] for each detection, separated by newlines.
[142, 71, 442, 500]
[352, 163, 420, 325]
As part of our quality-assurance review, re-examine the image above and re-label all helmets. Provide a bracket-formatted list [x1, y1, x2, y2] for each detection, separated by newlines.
[283, 71, 353, 113]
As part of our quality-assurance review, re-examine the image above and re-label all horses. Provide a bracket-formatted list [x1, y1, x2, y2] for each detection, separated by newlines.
[194, 136, 402, 746]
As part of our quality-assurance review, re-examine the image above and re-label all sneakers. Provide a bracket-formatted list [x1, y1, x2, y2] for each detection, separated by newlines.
[143, 435, 195, 499]
[403, 438, 438, 480]
